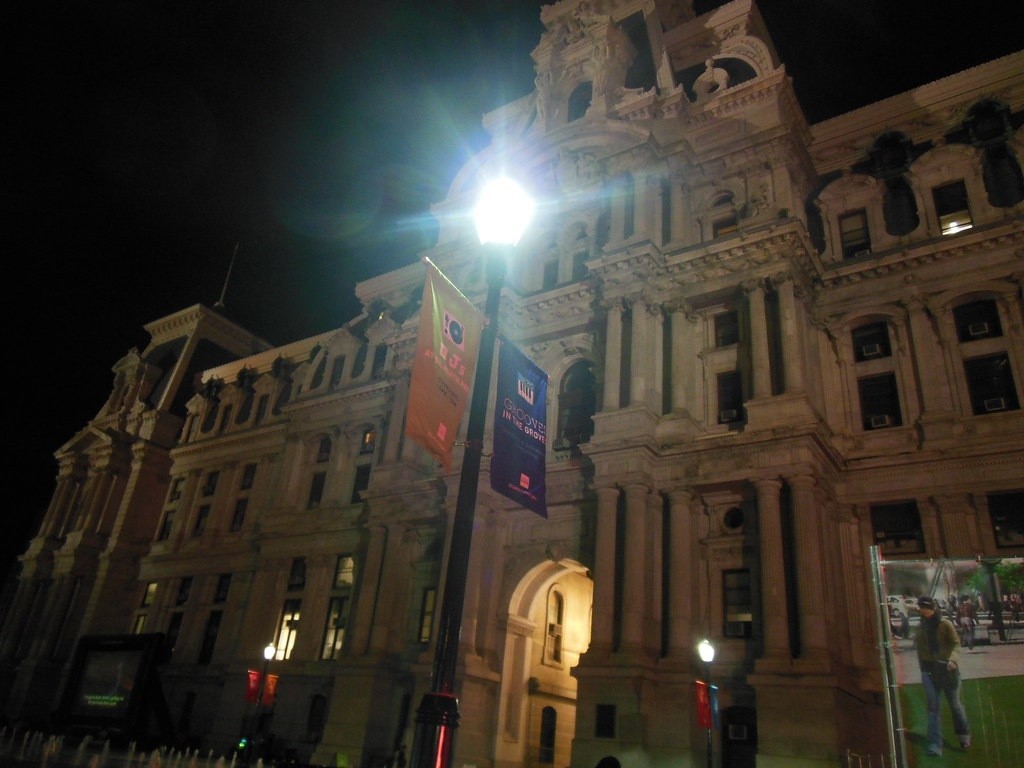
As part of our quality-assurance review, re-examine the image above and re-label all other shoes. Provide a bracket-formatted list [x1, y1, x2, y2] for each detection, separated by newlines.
[925, 749, 937, 757]
[961, 740, 970, 751]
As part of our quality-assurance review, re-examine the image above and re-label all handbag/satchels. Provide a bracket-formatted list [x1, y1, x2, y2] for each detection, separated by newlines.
[961, 617, 973, 626]
[934, 660, 958, 689]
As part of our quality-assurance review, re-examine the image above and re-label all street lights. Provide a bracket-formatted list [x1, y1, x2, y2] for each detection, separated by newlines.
[404, 172, 540, 768]
[237, 641, 279, 764]
[697, 636, 716, 768]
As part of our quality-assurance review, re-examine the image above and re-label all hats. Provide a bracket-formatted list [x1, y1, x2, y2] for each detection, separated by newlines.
[915, 598, 934, 609]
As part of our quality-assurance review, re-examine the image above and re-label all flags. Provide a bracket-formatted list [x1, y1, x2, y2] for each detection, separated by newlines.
[263, 675, 279, 704]
[695, 680, 721, 730]
[489, 334, 549, 520]
[246, 670, 261, 704]
[406, 251, 485, 477]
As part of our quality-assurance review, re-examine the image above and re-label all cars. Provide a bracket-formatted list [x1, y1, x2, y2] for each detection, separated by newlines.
[885, 593, 918, 618]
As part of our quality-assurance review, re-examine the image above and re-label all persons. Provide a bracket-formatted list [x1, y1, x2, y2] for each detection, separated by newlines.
[918, 593, 973, 756]
[1010, 592, 1022, 622]
[595, 756, 621, 768]
[934, 591, 988, 650]
[886, 593, 912, 640]
